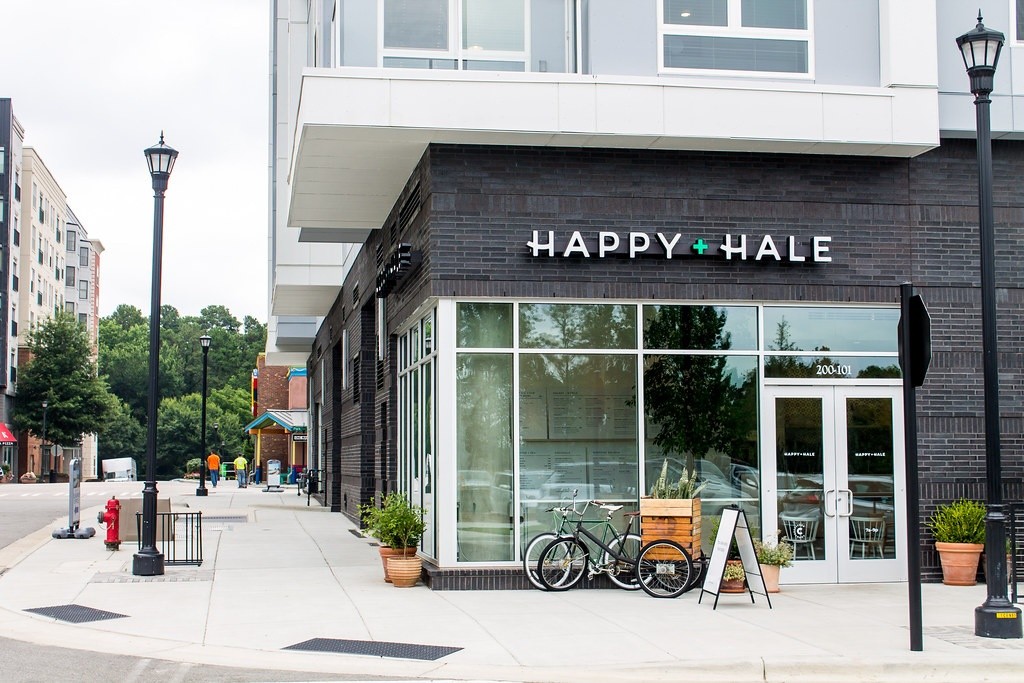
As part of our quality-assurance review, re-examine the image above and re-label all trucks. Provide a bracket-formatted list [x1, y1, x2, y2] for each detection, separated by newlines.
[101, 457, 137, 482]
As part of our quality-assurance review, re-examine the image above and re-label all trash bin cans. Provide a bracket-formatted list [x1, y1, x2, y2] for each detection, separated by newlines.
[218, 464, 227, 477]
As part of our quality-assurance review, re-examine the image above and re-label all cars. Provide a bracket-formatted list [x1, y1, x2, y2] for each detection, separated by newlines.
[654, 457, 737, 489]
[729, 463, 796, 508]
[538, 462, 759, 536]
[489, 470, 554, 524]
[457, 470, 493, 517]
[777, 472, 796, 508]
[778, 474, 895, 539]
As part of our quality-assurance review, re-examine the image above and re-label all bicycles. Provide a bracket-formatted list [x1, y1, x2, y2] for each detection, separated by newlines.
[523, 500, 654, 591]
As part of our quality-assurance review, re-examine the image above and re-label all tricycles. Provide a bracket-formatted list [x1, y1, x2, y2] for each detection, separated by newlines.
[537, 489, 710, 598]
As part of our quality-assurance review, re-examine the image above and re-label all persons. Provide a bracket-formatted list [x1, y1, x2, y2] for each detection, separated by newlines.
[207, 449, 221, 487]
[232, 452, 248, 488]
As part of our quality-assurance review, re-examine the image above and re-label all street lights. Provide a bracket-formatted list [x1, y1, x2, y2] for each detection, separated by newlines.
[213, 422, 219, 455]
[196, 330, 212, 496]
[132, 129, 180, 576]
[955, 9, 1024, 639]
[221, 440, 225, 462]
[40, 398, 48, 483]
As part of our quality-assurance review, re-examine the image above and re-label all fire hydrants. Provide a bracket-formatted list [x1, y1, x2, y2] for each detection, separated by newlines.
[97, 496, 122, 551]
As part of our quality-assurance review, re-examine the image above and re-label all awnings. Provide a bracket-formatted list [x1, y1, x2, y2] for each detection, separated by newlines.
[0, 422, 18, 446]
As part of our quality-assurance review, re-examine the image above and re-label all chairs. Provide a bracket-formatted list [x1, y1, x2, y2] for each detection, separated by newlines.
[849, 516, 887, 559]
[781, 515, 819, 560]
[280, 467, 291, 484]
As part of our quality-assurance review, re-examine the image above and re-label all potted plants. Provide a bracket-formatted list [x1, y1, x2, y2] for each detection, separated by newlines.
[983, 536, 1012, 584]
[637, 456, 710, 561]
[923, 496, 987, 586]
[752, 529, 794, 593]
[355, 490, 430, 588]
[707, 514, 756, 589]
